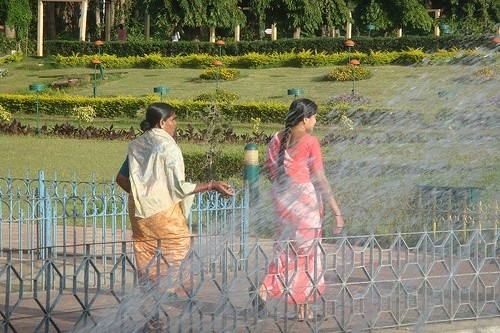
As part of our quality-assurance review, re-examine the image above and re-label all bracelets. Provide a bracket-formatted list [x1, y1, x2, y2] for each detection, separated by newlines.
[333, 214, 342, 216]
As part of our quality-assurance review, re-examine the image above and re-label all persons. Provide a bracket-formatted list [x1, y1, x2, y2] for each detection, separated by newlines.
[118, 24, 125, 41]
[116, 102, 239, 304]
[250, 98, 346, 323]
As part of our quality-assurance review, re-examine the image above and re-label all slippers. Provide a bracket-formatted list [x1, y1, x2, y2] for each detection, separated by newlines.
[297, 309, 327, 323]
[249, 284, 269, 320]
